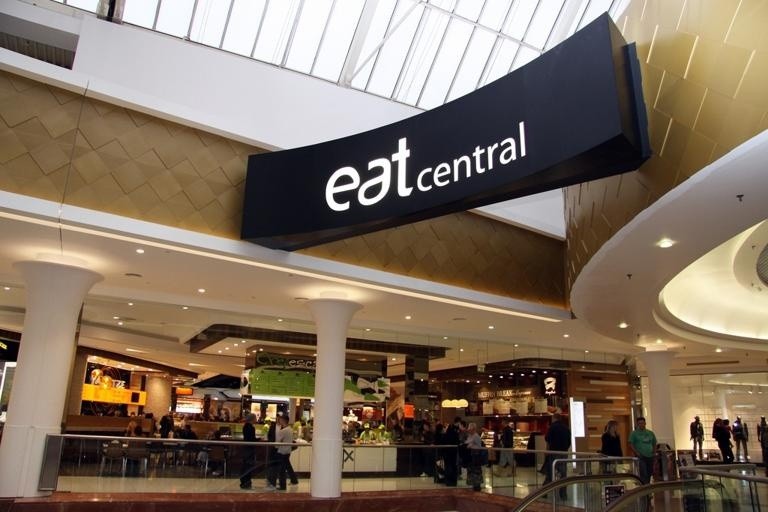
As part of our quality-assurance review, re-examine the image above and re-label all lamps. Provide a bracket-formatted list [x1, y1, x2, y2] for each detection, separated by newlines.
[441, 380, 469, 407]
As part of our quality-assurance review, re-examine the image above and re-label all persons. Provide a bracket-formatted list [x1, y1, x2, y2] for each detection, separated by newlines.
[601, 421, 623, 457]
[358, 416, 516, 491]
[628, 418, 657, 485]
[114, 408, 313, 492]
[690, 416, 705, 461]
[539, 414, 571, 501]
[712, 415, 768, 463]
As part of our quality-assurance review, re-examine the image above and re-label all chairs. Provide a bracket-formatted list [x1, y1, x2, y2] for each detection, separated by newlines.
[57, 418, 274, 480]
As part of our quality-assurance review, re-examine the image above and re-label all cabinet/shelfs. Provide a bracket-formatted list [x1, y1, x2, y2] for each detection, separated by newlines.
[480, 430, 542, 466]
